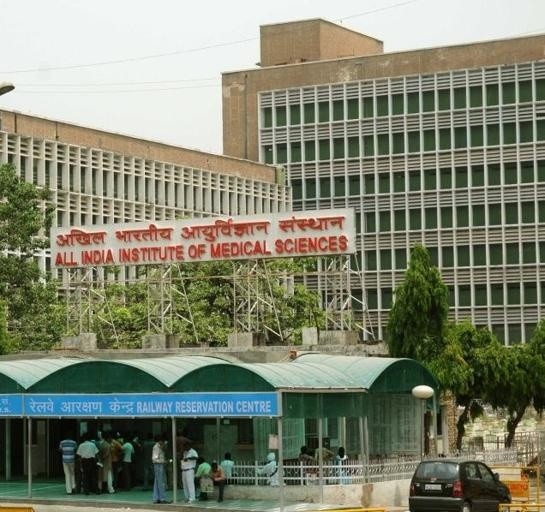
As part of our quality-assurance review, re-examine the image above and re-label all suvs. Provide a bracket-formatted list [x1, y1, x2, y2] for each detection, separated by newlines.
[408, 459, 511, 511]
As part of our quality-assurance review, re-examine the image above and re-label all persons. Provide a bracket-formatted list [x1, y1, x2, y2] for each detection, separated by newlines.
[258, 452, 286, 486]
[299, 442, 350, 485]
[59, 431, 234, 504]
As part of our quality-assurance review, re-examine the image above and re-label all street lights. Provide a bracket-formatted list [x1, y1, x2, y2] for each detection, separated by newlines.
[0, 80, 15, 97]
[412, 384, 434, 462]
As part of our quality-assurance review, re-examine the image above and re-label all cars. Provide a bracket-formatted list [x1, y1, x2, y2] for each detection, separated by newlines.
[520, 449, 545, 483]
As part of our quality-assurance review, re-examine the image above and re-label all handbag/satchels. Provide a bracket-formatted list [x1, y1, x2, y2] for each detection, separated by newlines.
[200, 478, 213, 493]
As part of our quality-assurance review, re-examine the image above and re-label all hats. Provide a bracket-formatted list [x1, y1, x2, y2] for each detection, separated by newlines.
[267, 453, 275, 462]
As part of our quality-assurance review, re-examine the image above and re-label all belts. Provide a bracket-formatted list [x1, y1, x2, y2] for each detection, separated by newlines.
[182, 469, 190, 472]
